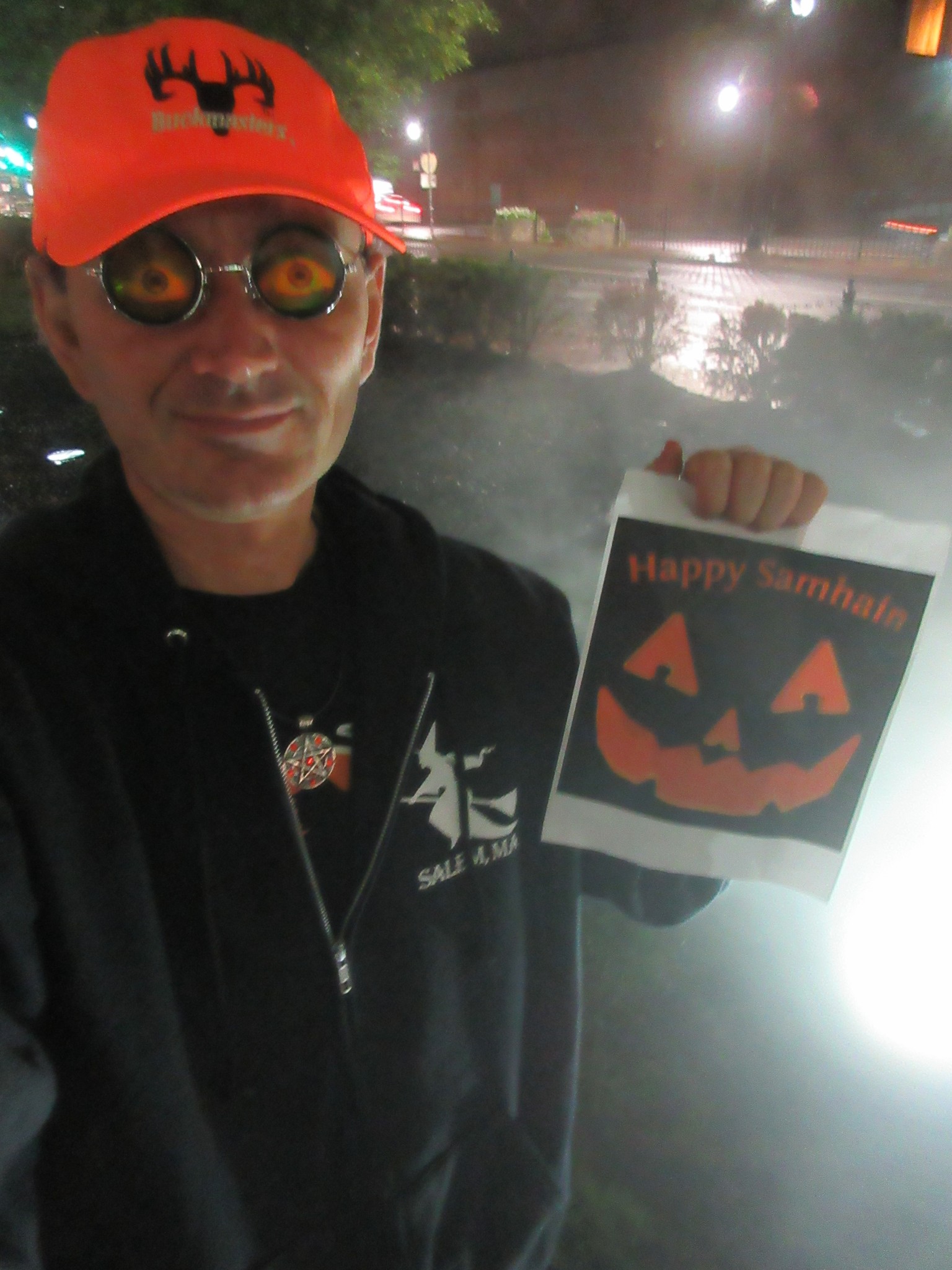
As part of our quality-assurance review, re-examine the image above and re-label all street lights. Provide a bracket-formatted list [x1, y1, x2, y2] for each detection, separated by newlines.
[719, 0, 816, 266]
[404, 111, 437, 238]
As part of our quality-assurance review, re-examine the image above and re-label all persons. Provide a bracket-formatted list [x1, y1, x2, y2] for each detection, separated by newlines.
[2, 17, 830, 1270]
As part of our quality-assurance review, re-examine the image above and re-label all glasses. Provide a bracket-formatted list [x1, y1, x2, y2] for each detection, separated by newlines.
[86, 221, 369, 327]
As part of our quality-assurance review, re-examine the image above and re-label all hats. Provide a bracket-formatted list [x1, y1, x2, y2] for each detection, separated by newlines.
[30, 17, 409, 268]
